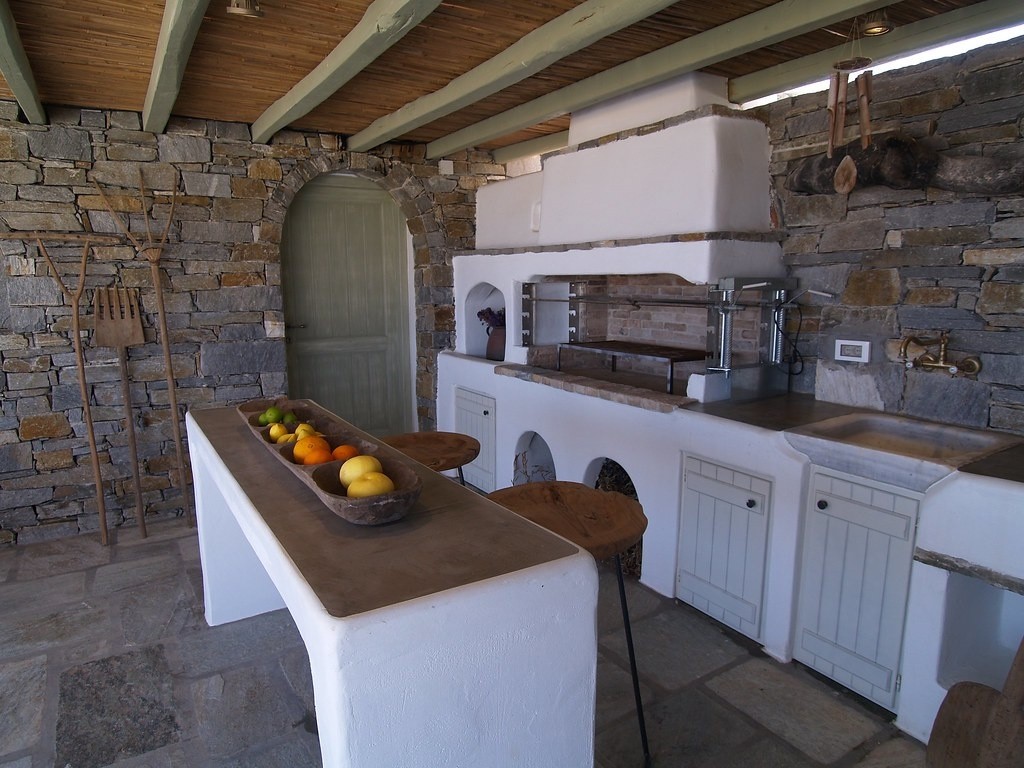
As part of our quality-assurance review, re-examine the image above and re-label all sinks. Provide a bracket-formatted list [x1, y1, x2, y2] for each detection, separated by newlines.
[782, 413, 1024, 493]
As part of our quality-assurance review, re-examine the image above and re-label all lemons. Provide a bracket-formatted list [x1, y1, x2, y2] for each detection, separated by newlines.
[268, 423, 319, 445]
[258, 406, 296, 426]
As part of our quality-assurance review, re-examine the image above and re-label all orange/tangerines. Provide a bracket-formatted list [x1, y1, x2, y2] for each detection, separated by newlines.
[293, 435, 359, 465]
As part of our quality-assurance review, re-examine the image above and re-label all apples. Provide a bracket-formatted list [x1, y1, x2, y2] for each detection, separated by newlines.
[338, 455, 394, 498]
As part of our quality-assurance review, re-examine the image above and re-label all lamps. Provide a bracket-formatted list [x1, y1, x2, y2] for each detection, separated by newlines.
[858, 7, 895, 37]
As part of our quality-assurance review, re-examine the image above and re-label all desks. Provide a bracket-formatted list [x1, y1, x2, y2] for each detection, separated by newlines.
[185, 398, 599, 768]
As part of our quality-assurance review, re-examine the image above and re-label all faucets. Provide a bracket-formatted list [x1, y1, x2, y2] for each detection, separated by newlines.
[897, 326, 951, 367]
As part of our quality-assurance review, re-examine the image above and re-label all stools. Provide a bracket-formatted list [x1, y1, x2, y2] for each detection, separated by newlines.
[375, 432, 480, 485]
[486, 481, 653, 768]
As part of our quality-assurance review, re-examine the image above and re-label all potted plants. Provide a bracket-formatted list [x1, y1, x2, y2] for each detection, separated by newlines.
[477, 307, 506, 361]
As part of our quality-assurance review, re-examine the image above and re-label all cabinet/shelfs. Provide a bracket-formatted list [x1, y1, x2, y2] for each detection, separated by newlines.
[455, 387, 496, 494]
[675, 450, 776, 646]
[791, 463, 927, 716]
